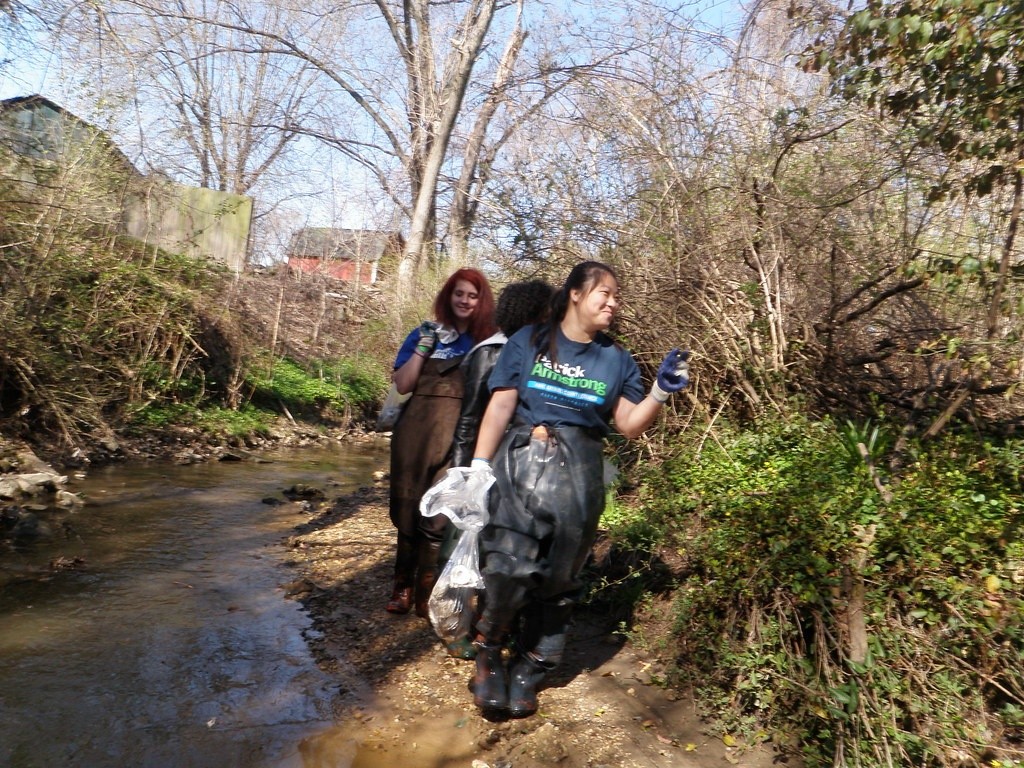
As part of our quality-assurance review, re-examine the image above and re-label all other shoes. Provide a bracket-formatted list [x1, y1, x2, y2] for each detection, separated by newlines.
[506, 617, 522, 648]
[441, 634, 477, 659]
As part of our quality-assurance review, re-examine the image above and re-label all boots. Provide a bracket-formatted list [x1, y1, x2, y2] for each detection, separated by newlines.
[387, 532, 450, 617]
[471, 640, 546, 715]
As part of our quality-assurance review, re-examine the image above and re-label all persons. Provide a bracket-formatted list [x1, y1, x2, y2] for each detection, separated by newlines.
[381, 266, 499, 617]
[453, 259, 693, 717]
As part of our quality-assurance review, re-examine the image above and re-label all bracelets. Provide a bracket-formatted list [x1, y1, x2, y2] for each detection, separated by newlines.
[414, 345, 431, 359]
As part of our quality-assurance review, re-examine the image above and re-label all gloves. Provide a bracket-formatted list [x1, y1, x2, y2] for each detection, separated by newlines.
[651, 348, 690, 403]
[418, 321, 439, 348]
[471, 458, 491, 477]
